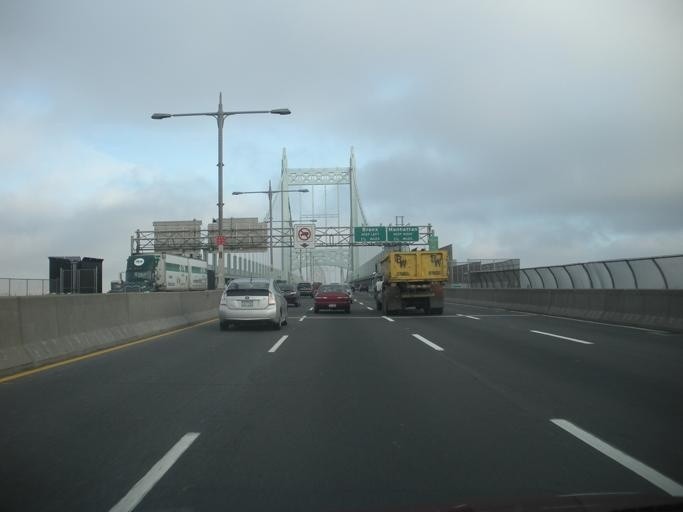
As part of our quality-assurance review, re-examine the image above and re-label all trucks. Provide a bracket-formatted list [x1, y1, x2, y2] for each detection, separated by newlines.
[368, 248, 451, 316]
[123, 251, 212, 293]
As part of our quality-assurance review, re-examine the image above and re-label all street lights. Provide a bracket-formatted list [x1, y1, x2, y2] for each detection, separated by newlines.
[226, 179, 319, 279]
[150, 106, 292, 291]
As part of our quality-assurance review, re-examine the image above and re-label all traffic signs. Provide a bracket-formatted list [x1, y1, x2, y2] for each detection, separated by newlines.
[386, 226, 419, 243]
[352, 225, 387, 243]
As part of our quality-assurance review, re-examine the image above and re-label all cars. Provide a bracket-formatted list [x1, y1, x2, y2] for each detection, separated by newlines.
[214, 277, 369, 334]
